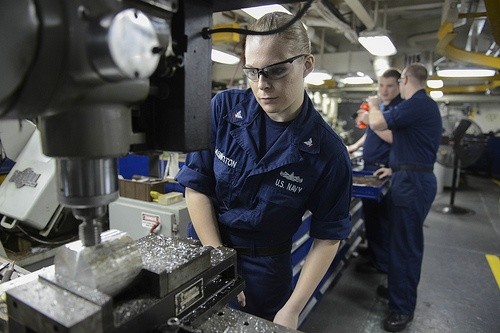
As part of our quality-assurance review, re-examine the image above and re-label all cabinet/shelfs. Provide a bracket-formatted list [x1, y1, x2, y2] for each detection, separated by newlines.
[108, 197, 191, 241]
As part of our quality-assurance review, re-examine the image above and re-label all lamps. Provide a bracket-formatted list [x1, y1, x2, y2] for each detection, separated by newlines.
[338, 49, 373, 85]
[304, 27, 332, 86]
[358, 4, 398, 57]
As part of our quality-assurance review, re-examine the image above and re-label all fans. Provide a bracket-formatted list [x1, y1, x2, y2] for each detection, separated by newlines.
[430, 115, 484, 216]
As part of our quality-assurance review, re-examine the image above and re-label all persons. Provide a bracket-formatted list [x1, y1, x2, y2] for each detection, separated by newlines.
[345, 56, 445, 332]
[173, 12, 353, 331]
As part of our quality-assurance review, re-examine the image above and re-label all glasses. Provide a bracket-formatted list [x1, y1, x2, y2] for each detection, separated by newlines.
[398, 77, 405, 83]
[241, 53, 308, 79]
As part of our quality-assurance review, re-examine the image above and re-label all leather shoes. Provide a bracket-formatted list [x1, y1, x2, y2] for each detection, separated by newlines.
[383, 311, 414, 331]
[376, 284, 390, 299]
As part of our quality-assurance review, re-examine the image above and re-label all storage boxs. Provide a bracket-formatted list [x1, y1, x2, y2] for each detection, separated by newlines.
[0, 126, 60, 230]
[352, 170, 391, 202]
[118, 178, 185, 205]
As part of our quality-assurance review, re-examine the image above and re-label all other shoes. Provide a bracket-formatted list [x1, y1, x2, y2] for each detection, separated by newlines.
[355, 261, 382, 275]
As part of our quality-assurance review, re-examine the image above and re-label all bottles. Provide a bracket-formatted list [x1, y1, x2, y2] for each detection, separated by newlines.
[358, 100, 369, 128]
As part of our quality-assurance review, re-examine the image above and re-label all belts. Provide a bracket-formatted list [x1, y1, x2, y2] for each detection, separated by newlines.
[392, 165, 432, 172]
